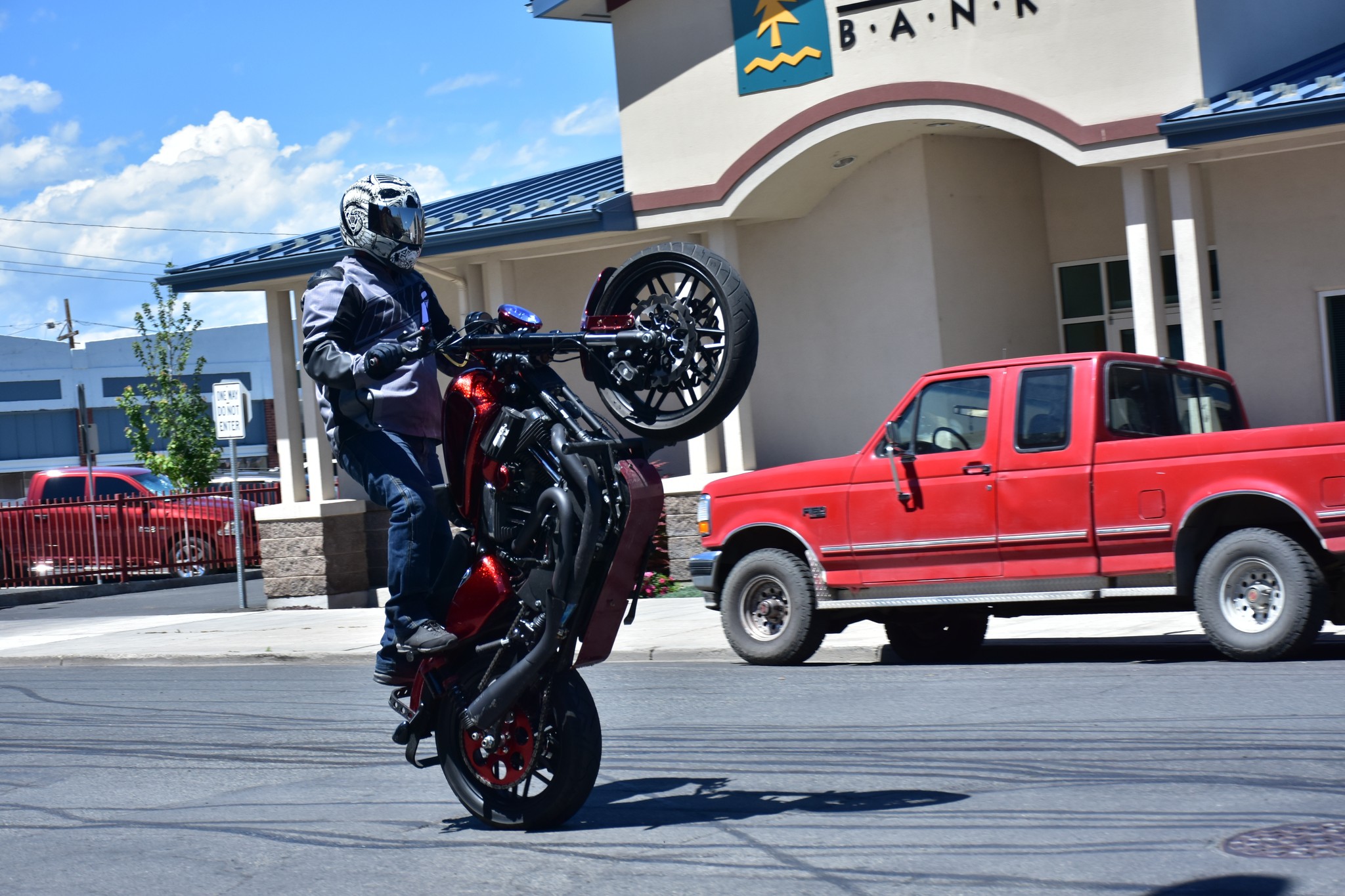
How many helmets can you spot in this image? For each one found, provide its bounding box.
[339,174,423,268]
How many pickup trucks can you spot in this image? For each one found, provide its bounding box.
[683,349,1345,664]
[0,466,273,582]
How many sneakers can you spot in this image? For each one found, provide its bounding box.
[373,668,415,686]
[396,621,458,653]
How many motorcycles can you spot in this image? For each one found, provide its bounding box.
[387,238,761,833]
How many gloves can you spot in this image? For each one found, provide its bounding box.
[365,341,407,381]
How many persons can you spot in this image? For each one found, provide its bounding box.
[299,173,493,690]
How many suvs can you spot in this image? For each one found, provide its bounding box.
[183,467,339,507]
